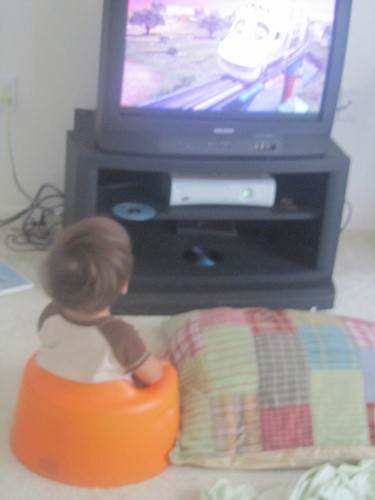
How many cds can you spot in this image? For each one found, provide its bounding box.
[184,245,224,267]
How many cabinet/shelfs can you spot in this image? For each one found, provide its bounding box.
[61,107,351,317]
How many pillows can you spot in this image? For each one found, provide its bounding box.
[165,307,374,467]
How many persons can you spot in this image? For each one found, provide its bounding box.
[33,217,172,390]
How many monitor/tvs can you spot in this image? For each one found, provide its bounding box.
[95,0,352,158]
